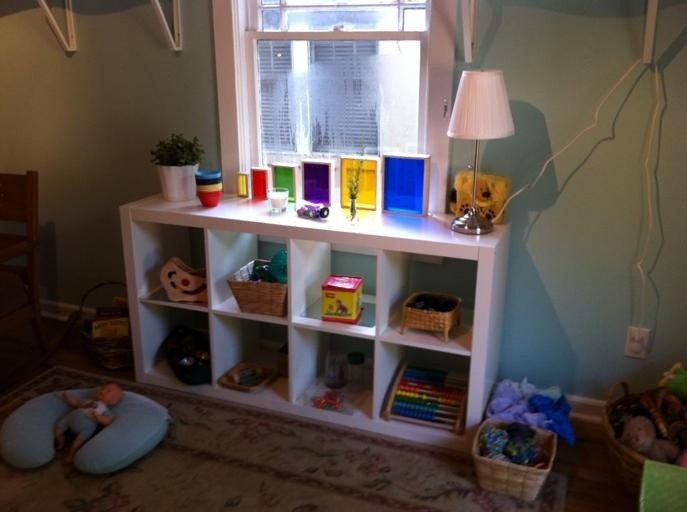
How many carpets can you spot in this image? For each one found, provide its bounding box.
[0,367,569,512]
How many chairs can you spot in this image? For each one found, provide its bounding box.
[1,170,55,393]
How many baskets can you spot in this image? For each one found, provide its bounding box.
[602,381,687,497]
[78,282,133,369]
[472,419,558,501]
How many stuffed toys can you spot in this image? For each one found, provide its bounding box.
[654,362,687,395]
[623,416,679,462]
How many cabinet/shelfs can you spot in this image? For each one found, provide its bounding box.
[120,190,512,455]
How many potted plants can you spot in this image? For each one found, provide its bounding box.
[149,134,206,201]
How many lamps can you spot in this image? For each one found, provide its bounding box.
[446,70,516,235]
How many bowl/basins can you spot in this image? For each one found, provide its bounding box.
[195,171,223,207]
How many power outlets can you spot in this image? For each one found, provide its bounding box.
[624,326,651,360]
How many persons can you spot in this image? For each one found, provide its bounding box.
[55,382,124,462]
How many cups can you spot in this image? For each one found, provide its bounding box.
[324,354,347,388]
[267,188,289,214]
